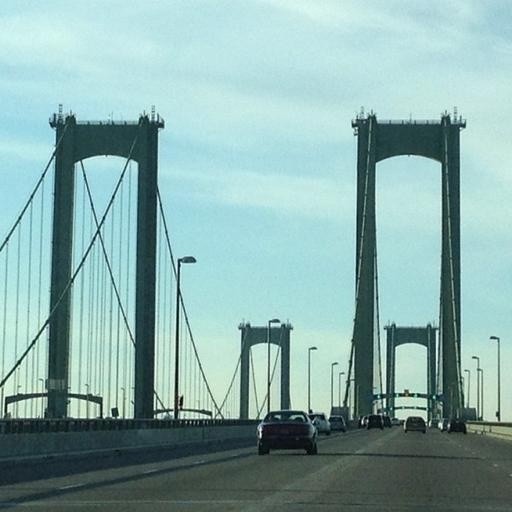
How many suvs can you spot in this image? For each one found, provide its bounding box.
[306,412,332,435]
[326,415,346,432]
[358,414,400,430]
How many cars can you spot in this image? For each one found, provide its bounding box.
[402,416,426,434]
[428,418,440,428]
[256,409,318,454]
[446,419,467,434]
[437,417,448,432]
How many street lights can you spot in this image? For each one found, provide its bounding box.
[307,347,317,412]
[463,369,470,409]
[330,362,339,409]
[173,256,197,421]
[471,355,479,421]
[489,335,500,422]
[14,379,158,422]
[347,378,356,415]
[266,317,280,414]
[476,369,483,421]
[338,371,345,407]
